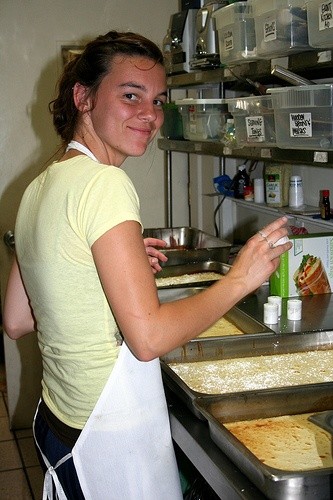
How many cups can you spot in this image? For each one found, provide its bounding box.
[253,179,264,203]
[264,304,278,324]
[287,300,301,321]
[268,296,282,316]
[288,175,304,208]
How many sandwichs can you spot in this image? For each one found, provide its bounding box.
[294,253,331,296]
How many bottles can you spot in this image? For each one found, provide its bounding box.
[233,164,250,199]
[162,28,172,74]
[320,191,331,221]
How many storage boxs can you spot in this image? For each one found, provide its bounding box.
[161,0,333,151]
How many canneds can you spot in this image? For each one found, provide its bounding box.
[264,161,289,207]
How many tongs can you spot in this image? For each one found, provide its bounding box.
[270,64,317,85]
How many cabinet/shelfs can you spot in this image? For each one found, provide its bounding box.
[158,48,333,167]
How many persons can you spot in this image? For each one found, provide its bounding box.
[0,30,295,499]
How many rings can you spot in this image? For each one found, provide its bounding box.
[264,237,273,247]
[257,230,266,238]
